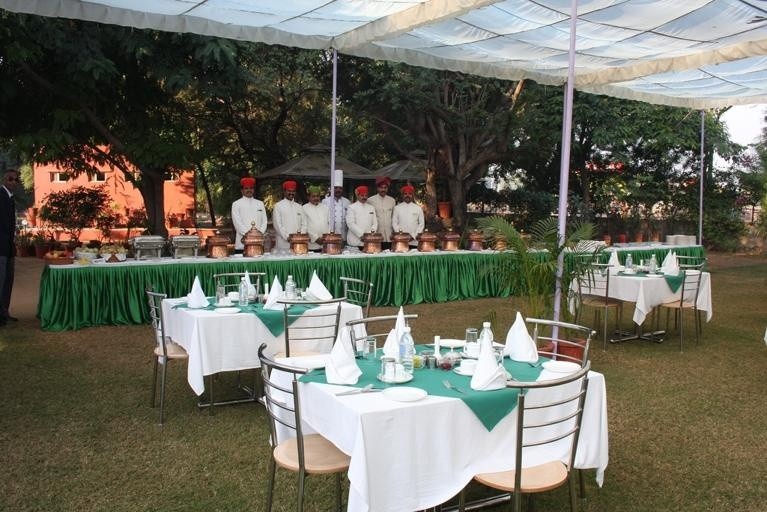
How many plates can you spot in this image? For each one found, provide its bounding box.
[379,386,427,402]
[378,373,414,384]
[437,338,465,349]
[272,353,332,371]
[539,360,582,374]
[188,294,243,314]
[454,366,473,376]
[623,271,638,275]
[646,274,664,278]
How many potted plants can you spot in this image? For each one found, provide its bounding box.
[480,211,597,359]
[538,241,610,362]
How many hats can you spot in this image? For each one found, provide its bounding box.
[240,177,256,186]
[334,169,344,188]
[306,186,320,194]
[401,185,416,193]
[283,181,297,190]
[355,186,368,195]
[376,176,391,187]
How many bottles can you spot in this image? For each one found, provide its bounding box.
[626,254,633,268]
[650,255,657,274]
[239,277,251,306]
[399,326,415,374]
[478,321,494,356]
[285,275,295,301]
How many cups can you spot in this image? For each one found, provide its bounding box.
[427,356,437,369]
[380,357,397,386]
[461,359,476,371]
[466,327,478,344]
[493,347,505,366]
[363,338,377,360]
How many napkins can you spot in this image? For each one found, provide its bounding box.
[500,311,538,363]
[325,328,363,384]
[383,306,417,358]
[471,329,508,391]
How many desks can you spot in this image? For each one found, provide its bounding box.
[32,240,707,333]
[258,338,611,511]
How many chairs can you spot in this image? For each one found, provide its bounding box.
[457,359,592,511]
[144,271,376,426]
[345,314,419,352]
[257,342,353,511]
[526,317,596,368]
[276,296,348,358]
[575,249,714,352]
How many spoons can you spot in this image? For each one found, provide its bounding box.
[335,383,374,396]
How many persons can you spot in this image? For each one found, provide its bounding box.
[230,175,269,257]
[301,184,332,253]
[366,175,396,251]
[390,184,426,250]
[1,169,19,327]
[321,186,351,249]
[271,179,309,250]
[345,185,378,252]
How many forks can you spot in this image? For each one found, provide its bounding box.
[439,379,467,395]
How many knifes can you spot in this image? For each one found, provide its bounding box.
[336,389,383,397]
